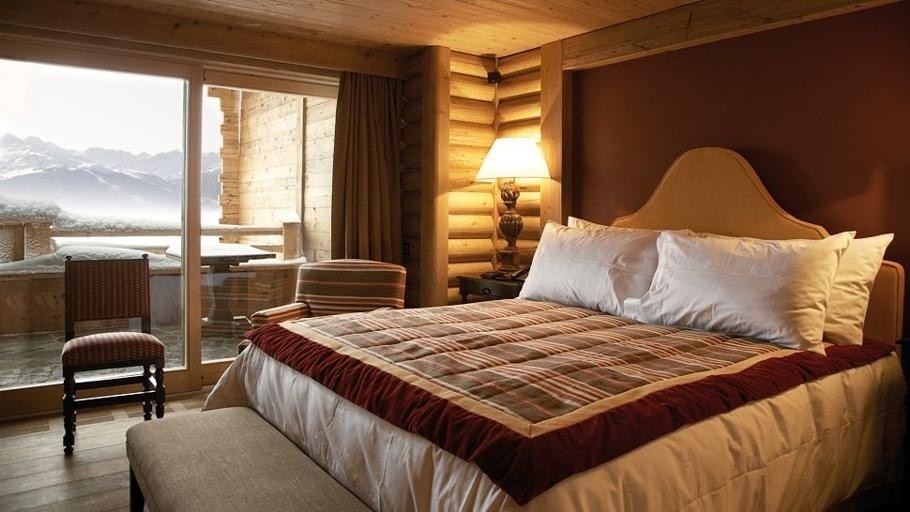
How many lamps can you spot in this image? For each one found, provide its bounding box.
[474,137,552,281]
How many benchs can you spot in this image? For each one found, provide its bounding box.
[123,407,376,511]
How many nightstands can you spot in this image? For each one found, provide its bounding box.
[456,274,527,302]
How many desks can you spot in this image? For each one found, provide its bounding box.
[166,242,276,337]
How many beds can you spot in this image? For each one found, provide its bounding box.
[243,146,904,512]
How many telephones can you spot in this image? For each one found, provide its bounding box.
[511,267,530,282]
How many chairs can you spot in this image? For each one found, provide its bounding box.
[62,254,166,459]
[236,258,407,356]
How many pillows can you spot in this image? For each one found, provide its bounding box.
[518,215,693,320]
[624,229,893,357]
